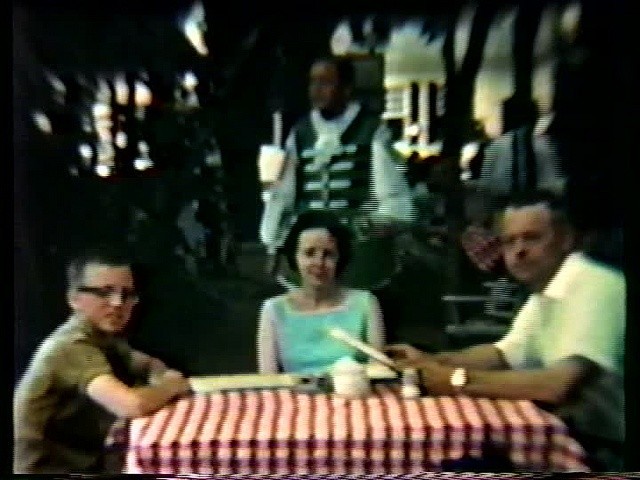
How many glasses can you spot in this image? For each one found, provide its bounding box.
[75,282,141,301]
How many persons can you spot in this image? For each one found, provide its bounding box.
[13,252,191,474]
[255,211,385,375]
[259,55,420,359]
[375,189,627,472]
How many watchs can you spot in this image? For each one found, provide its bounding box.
[449,367,469,397]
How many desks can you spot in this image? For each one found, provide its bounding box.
[123,382,591,480]
